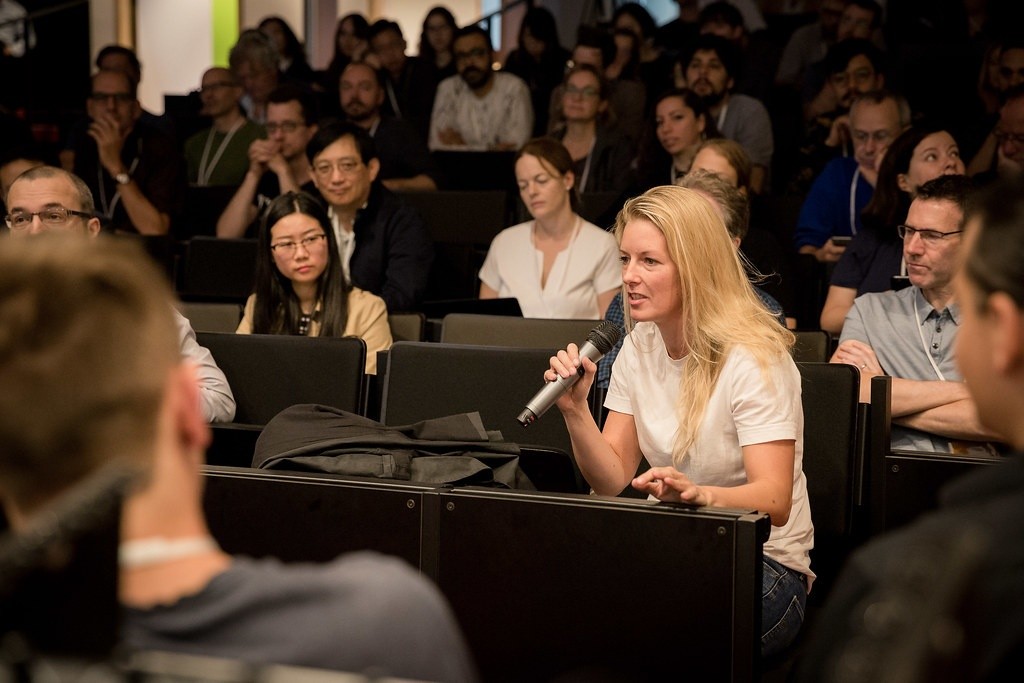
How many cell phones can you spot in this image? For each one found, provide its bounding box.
[832,236,852,246]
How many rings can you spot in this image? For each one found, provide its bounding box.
[860,364,866,370]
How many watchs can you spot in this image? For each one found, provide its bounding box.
[114,173,132,185]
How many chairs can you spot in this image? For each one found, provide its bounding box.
[130,64,1011,683]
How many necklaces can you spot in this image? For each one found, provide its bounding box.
[118,536,215,566]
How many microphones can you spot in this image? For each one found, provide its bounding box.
[516,321,623,427]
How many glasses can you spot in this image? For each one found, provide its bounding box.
[90,92,135,107]
[563,85,599,99]
[897,224,965,244]
[264,120,307,134]
[197,82,236,95]
[5,206,94,232]
[269,233,328,261]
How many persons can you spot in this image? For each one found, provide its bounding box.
[59,0,1024,389]
[795,174,1024,683]
[5,166,236,422]
[829,173,1005,458]
[0,226,471,683]
[544,184,815,650]
